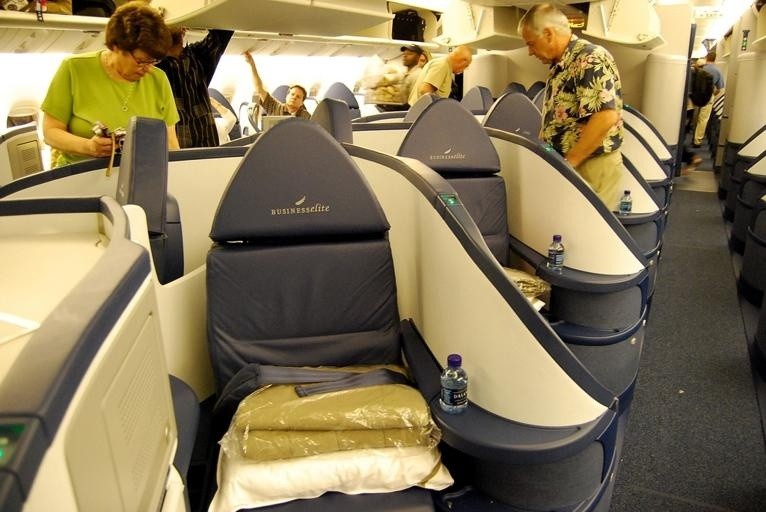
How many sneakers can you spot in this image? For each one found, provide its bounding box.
[692,142,701,149]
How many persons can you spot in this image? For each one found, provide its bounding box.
[153,25,220,149]
[681,52,725,171]
[517,2,624,213]
[207,87,241,141]
[40,0,181,169]
[240,49,312,122]
[375,45,472,112]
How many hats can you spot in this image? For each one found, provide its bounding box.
[400,43,424,56]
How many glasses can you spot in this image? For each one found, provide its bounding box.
[129,49,163,66]
[419,58,426,62]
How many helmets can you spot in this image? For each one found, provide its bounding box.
[695,57,706,67]
[422,47,433,62]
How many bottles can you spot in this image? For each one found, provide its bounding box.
[619,189,634,217]
[546,234,565,276]
[438,353,468,415]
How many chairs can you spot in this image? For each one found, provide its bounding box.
[104,83,675,512]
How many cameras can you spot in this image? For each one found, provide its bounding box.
[112,128,127,147]
[91,120,108,138]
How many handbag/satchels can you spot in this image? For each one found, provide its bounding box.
[391,7,427,42]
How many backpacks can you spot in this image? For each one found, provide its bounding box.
[689,68,715,109]
